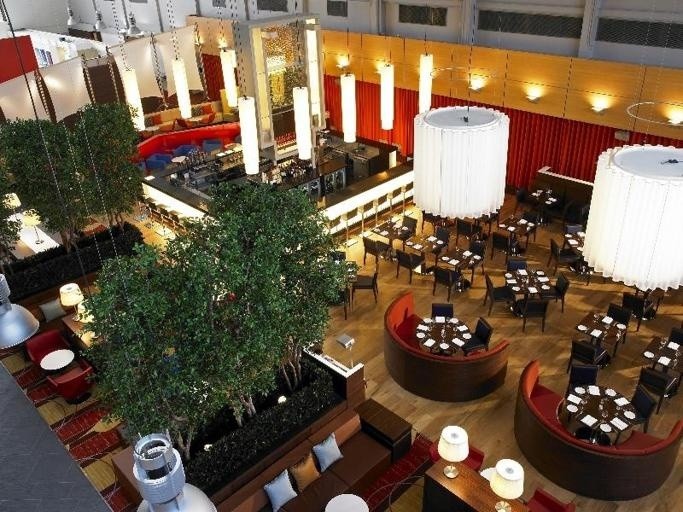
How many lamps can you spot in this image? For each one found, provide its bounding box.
[0,1,217,512]
[2,193,23,230]
[23,209,44,244]
[78,299,101,337]
[490,459,524,512]
[438,425,469,479]
[0,273,40,349]
[60,283,84,322]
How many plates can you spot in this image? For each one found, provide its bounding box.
[375,215,585,295]
[577,311,628,340]
[642,333,680,369]
[417,316,471,352]
[564,384,636,433]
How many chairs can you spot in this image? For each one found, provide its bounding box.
[526,488,576,512]
[463,446,485,472]
[47,359,96,405]
[25,328,70,376]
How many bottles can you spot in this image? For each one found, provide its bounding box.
[173,147,209,167]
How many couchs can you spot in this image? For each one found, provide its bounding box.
[514,360,683,501]
[217,398,412,512]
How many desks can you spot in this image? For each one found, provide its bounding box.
[40,349,75,375]
[325,494,369,512]
[425,458,531,512]
[61,313,109,352]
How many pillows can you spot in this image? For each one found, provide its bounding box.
[39,298,66,323]
[263,433,344,512]
